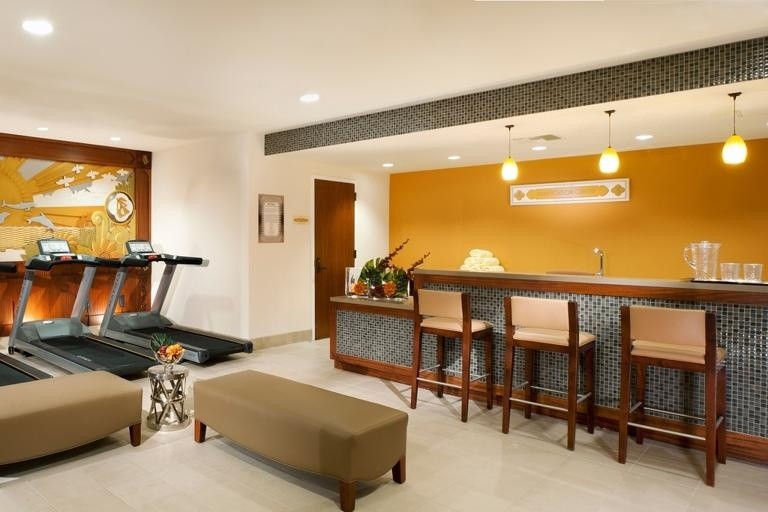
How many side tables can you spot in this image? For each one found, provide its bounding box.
[148,365,190,433]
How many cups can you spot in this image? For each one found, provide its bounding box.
[744,264,762,283]
[720,263,738,282]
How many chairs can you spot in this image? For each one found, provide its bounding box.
[501,296,596,450]
[411,288,494,422]
[618,305,726,488]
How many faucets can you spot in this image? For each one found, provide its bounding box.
[595,249,604,275]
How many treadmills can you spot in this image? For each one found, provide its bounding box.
[7,238,162,375]
[97,240,253,365]
[0,250,54,387]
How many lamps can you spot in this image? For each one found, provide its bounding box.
[722,93,748,166]
[501,125,519,181]
[599,110,620,174]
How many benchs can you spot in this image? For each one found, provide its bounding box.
[0,371,143,466]
[193,369,408,512]
